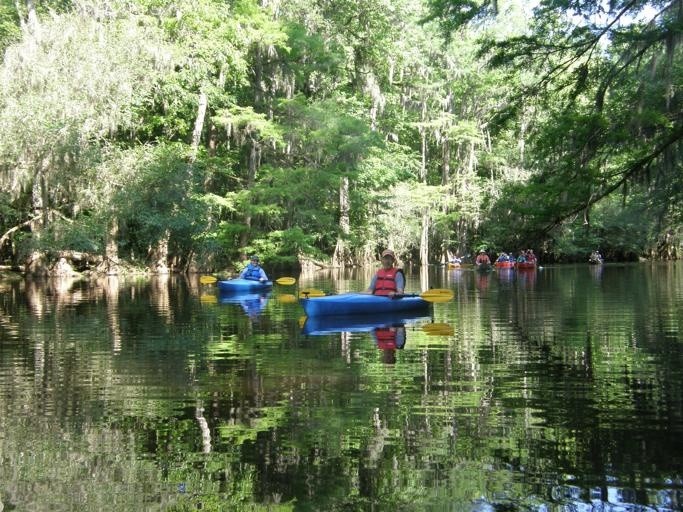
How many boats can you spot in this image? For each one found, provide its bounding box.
[218,278,272,292]
[473,258,538,272]
[447,262,460,268]
[216,293,274,314]
[297,293,429,317]
[589,257,601,264]
[298,314,430,336]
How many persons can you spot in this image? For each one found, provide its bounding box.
[589,250,603,265]
[448,256,462,263]
[475,249,537,264]
[240,256,269,283]
[366,249,405,299]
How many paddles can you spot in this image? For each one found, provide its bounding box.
[200,276,296,286]
[299,288,454,303]
[404,323,457,337]
[202,294,297,303]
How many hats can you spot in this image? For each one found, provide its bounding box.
[252,256,259,260]
[382,249,394,258]
[479,249,485,253]
